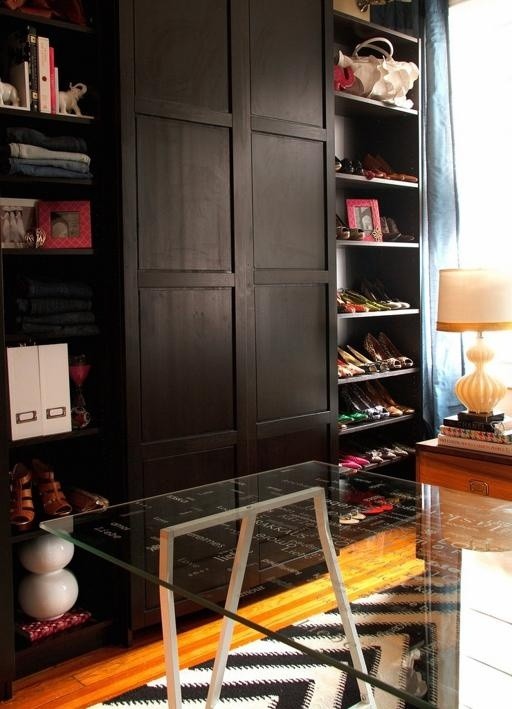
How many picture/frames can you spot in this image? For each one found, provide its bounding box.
[346,199,382,242]
[37,201,93,249]
[0,198,40,249]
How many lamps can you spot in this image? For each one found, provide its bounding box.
[437,269,512,423]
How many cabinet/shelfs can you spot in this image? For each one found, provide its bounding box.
[332,8,426,548]
[0,8,132,702]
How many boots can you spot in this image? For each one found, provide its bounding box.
[380,216,415,242]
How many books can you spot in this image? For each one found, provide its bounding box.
[0,24,61,115]
[443,411,512,433]
[437,434,512,457]
[438,424,512,445]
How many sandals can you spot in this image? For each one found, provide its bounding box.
[334,332,414,378]
[9,459,73,526]
[336,215,364,240]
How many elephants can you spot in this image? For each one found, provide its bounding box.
[58,81,88,117]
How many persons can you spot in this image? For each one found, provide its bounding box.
[1,210,11,243]
[9,211,20,243]
[15,210,27,242]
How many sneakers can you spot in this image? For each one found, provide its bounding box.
[335,154,418,182]
[340,442,416,469]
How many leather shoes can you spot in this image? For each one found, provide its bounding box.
[336,277,411,313]
[338,380,416,425]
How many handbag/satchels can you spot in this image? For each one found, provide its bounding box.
[334,37,419,109]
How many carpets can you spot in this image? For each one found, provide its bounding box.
[88,574,458,709]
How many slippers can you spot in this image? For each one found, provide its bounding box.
[339,493,399,525]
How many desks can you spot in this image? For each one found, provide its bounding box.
[39,460,512,709]
[414,437,512,577]
